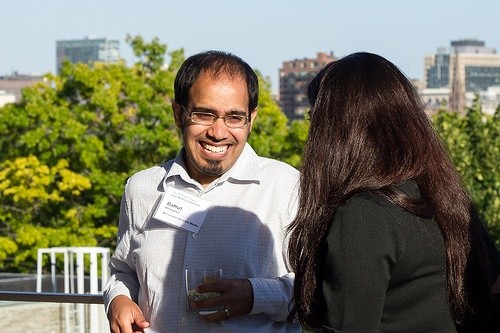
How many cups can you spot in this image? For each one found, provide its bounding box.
[185,270,223,311]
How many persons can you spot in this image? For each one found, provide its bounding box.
[284,52,500,333]
[103,50,303,333]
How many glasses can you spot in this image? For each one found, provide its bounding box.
[178,98,252,129]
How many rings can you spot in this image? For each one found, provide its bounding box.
[223,308,230,317]
